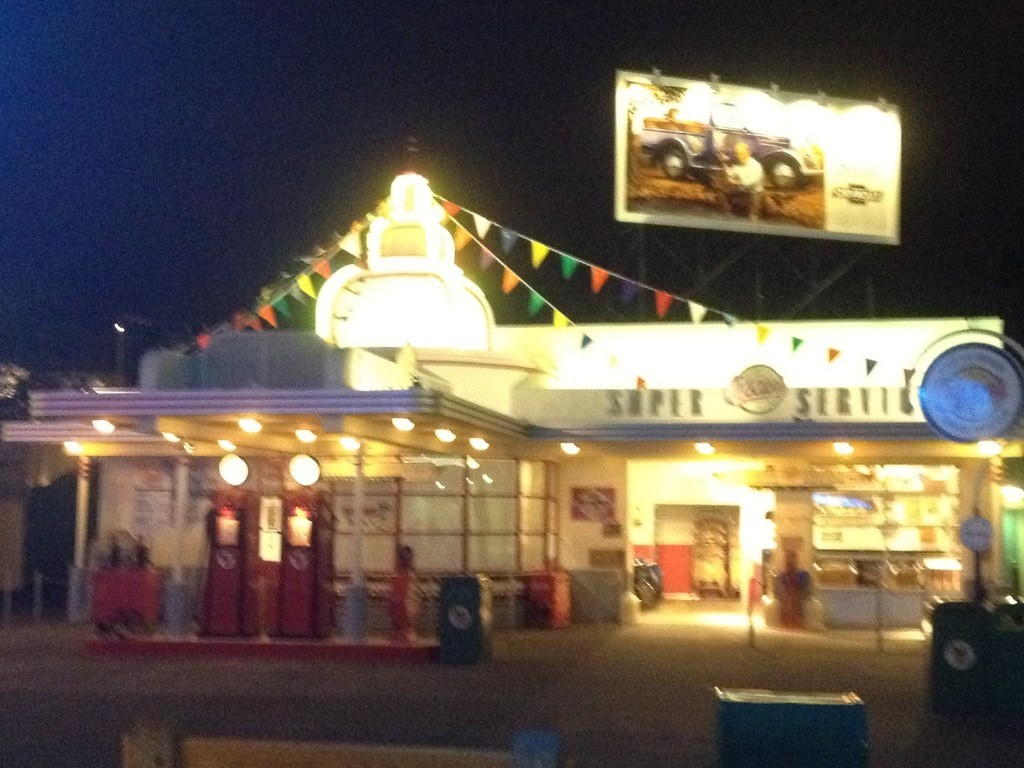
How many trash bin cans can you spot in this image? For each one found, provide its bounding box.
[927,602,993,714]
[438,574,492,667]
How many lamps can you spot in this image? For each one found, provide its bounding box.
[692,441,716,456]
[831,441,856,457]
[63,416,489,486]
[559,440,581,456]
